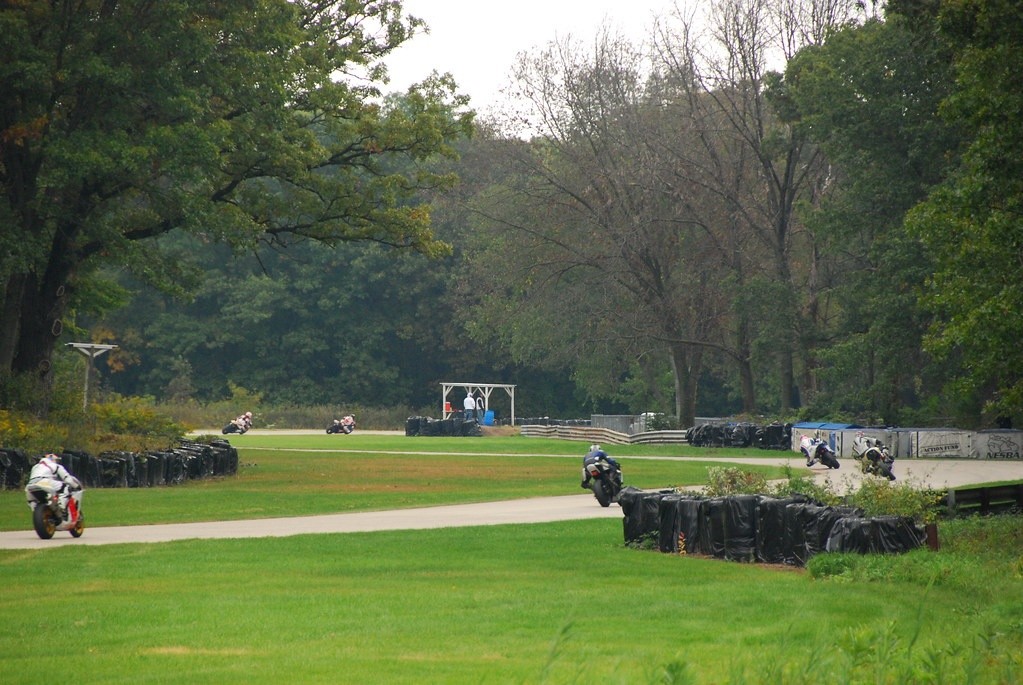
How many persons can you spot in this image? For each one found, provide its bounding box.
[800,435,836,457]
[25,454,81,512]
[476,393,484,425]
[852,430,889,463]
[463,393,475,420]
[239,412,252,429]
[581,444,619,487]
[342,414,355,433]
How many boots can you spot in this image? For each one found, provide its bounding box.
[28,500,37,511]
[57,497,69,516]
[813,458,819,463]
[614,469,622,486]
[825,445,835,454]
[583,483,593,489]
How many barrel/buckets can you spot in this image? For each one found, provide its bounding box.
[485,410,495,426]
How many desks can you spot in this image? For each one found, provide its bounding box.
[442,411,465,419]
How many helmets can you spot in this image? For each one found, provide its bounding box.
[589,445,600,450]
[855,430,864,437]
[45,454,61,464]
[246,412,252,419]
[351,414,355,418]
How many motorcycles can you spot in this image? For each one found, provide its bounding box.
[222,418,252,434]
[326,420,356,434]
[26,475,84,539]
[586,461,624,507]
[862,444,896,481]
[815,443,841,469]
